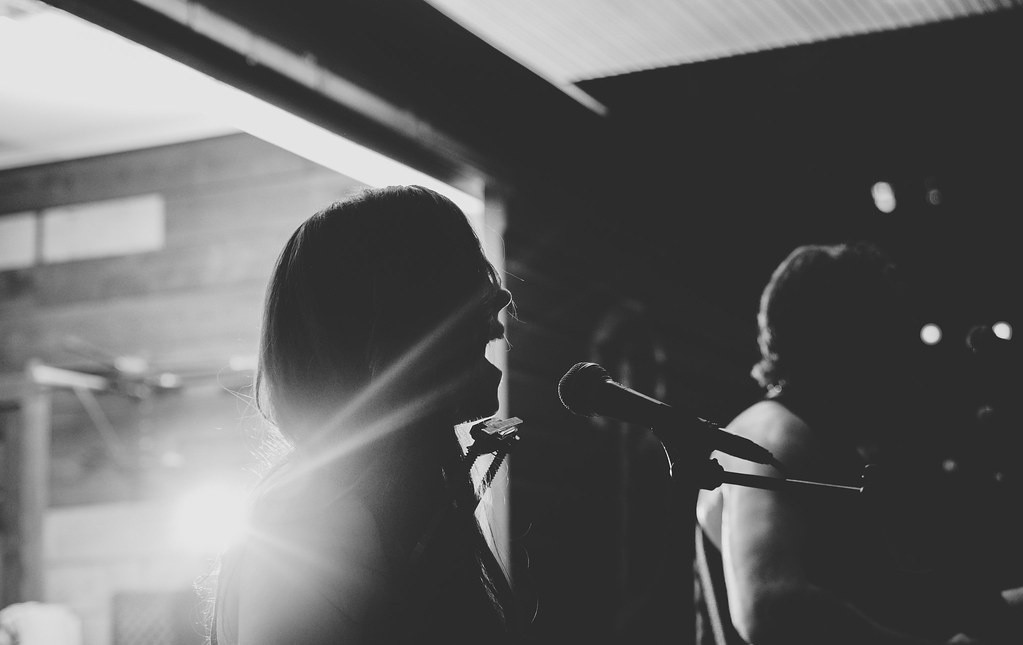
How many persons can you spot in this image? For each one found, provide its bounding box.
[210,186,519,644]
[694,241,1023,645]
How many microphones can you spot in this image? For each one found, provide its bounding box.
[557,362,776,465]
[967,325,999,357]
